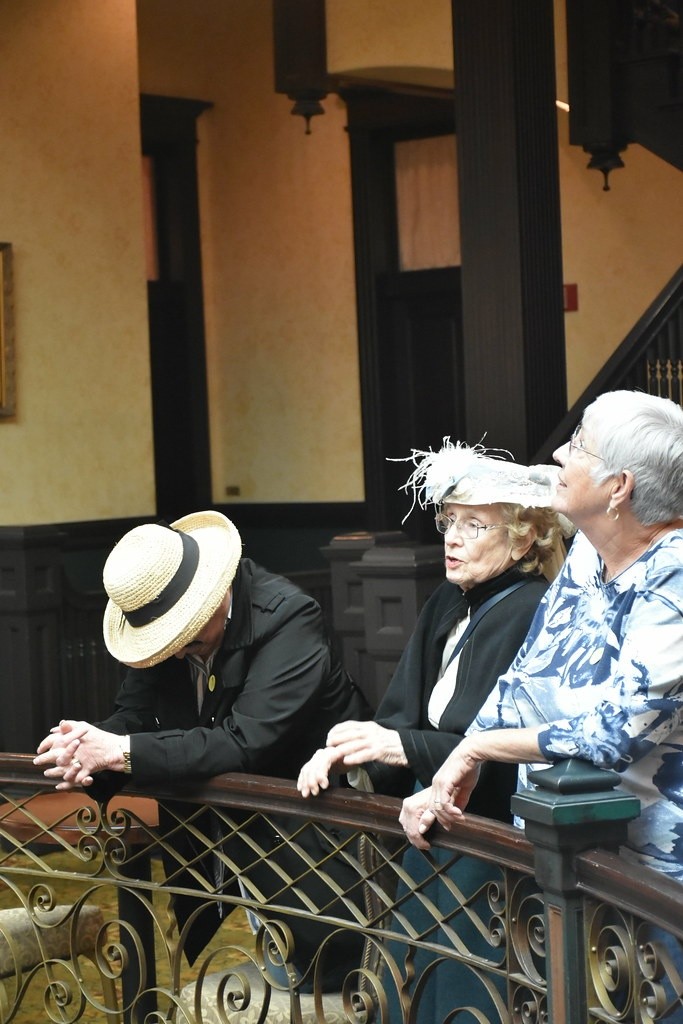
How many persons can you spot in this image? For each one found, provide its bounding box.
[399,390,683,1024]
[297,442,576,1024]
[33,511,375,991]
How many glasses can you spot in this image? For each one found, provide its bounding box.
[185,640,203,648]
[569,425,633,500]
[434,513,521,539]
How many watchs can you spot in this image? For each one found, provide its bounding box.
[122,735,132,773]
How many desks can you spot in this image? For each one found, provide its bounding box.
[0,792,160,1024]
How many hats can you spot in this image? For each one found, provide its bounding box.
[102,509,243,669]
[386,434,577,540]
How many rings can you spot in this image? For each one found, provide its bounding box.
[73,760,80,764]
[434,800,439,803]
